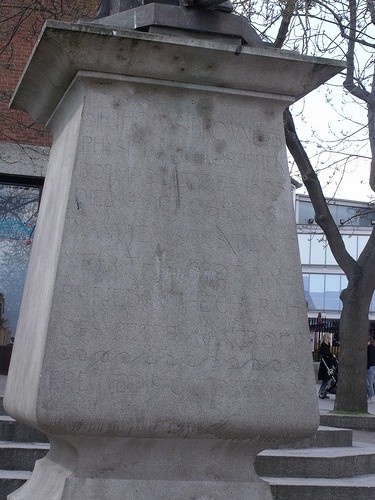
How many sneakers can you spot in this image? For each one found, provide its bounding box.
[367,395,373,403]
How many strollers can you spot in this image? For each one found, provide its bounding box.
[320,354,339,399]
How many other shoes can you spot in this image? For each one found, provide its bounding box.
[319,394,330,399]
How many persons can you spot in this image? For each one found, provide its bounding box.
[365,335,375,406]
[317,333,338,401]
